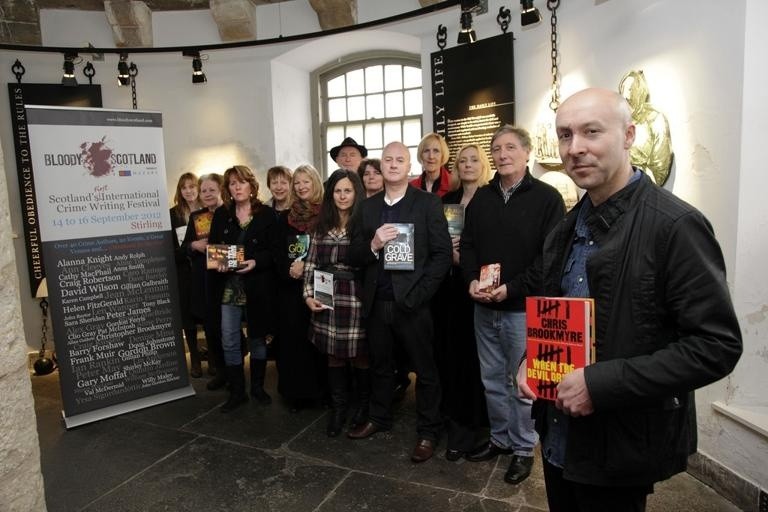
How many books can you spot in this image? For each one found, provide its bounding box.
[443,203,465,239]
[524,296,591,402]
[477,262,501,293]
[538,296,596,366]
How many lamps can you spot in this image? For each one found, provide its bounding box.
[54,43,211,92]
[449,1,544,45]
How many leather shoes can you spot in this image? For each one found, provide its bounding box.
[190,366,202,377]
[348,420,385,439]
[465,441,513,462]
[504,456,534,484]
[411,438,434,461]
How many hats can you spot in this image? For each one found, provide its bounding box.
[330,137,368,161]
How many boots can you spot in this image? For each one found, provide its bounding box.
[250,358,271,403]
[207,359,248,412]
[352,374,372,426]
[325,366,345,437]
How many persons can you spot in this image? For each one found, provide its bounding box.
[348,142,453,465]
[515,86,743,512]
[205,168,273,414]
[460,125,568,486]
[440,143,490,461]
[409,132,454,197]
[329,137,368,174]
[170,160,384,375]
[304,170,367,438]
